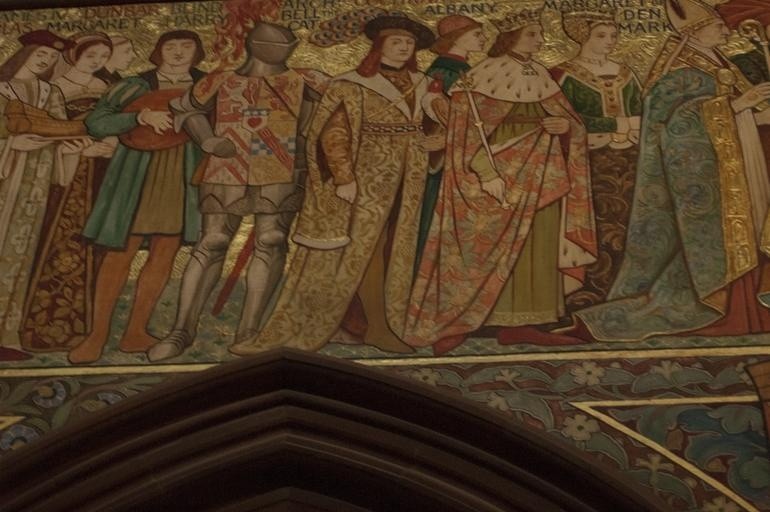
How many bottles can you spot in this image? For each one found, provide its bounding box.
[118,88,191,152]
[664,1,722,38]
[17,30,114,66]
[363,9,616,51]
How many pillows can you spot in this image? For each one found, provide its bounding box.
[244,22,300,65]
